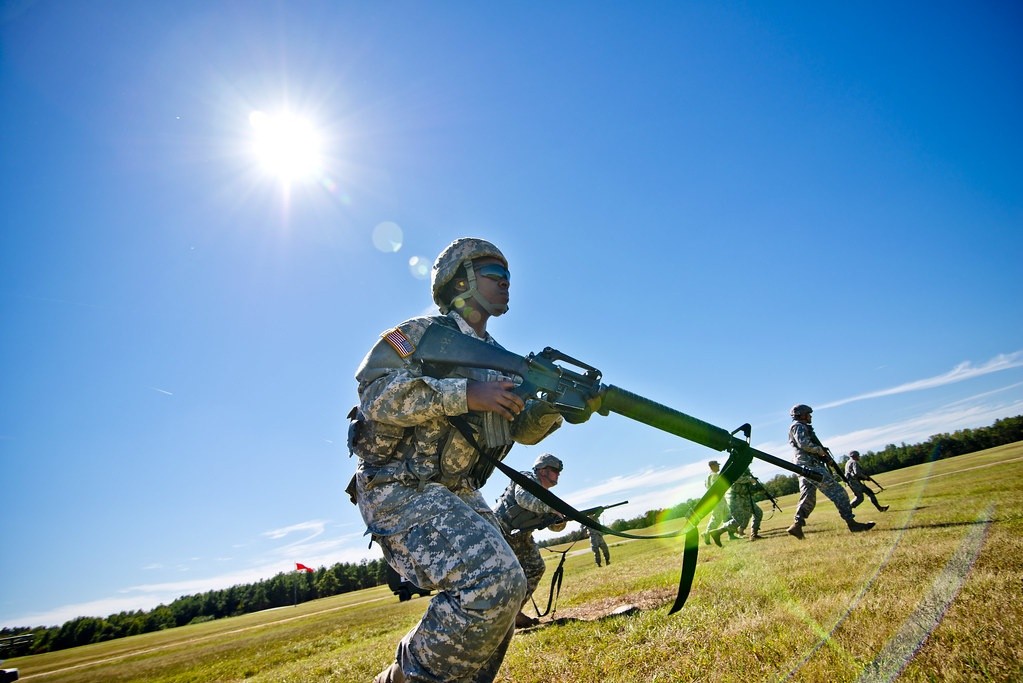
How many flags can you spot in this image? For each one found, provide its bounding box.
[296,563,313,572]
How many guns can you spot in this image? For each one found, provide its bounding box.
[808,430,850,487]
[863,474,885,490]
[748,475,784,514]
[509,501,629,535]
[412,323,826,485]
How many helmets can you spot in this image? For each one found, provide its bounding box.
[431,238,508,306]
[850,451,859,457]
[531,454,562,470]
[790,404,813,417]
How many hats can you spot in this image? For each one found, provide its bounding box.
[709,461,720,466]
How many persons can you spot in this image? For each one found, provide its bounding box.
[348,238,563,683]
[492,454,568,629]
[845,451,889,513]
[786,405,877,540]
[701,458,763,548]
[586,509,610,569]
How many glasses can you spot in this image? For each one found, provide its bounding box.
[472,264,510,282]
[542,468,560,474]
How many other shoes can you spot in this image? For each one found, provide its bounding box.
[374,660,407,683]
[878,505,889,512]
[846,519,876,532]
[701,530,712,546]
[730,532,743,540]
[786,522,805,540]
[597,563,601,567]
[739,527,746,536]
[606,560,610,565]
[750,528,761,541]
[515,611,539,629]
[709,525,727,547]
